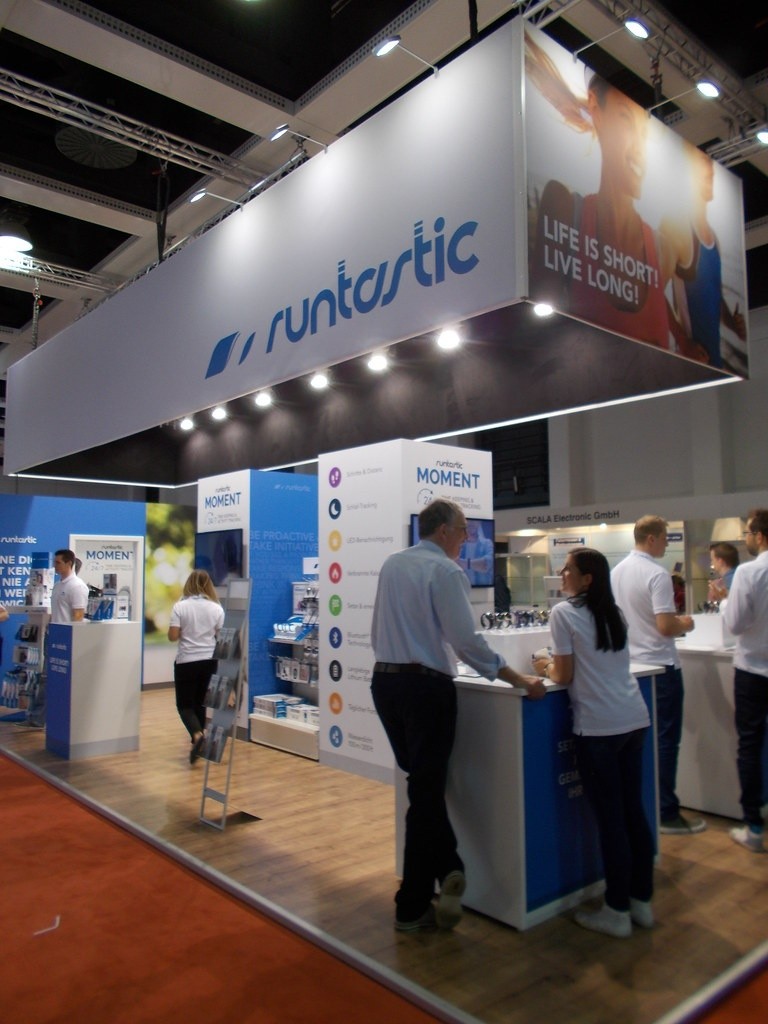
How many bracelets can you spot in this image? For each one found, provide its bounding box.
[544,662,551,677]
[467,558,470,569]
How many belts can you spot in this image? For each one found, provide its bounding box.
[373,662,452,680]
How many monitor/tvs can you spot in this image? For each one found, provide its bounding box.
[410,513,495,587]
[195,529,243,585]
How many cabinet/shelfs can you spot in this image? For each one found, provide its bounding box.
[1,606,49,728]
[249,579,320,761]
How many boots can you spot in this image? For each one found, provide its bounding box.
[632,899,654,927]
[575,902,632,938]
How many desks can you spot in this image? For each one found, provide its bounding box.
[395,613,743,932]
[45,620,142,760]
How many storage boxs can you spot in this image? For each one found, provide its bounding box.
[253,694,320,726]
[25,551,55,605]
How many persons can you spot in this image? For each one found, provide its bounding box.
[534,65,669,349]
[611,514,705,834]
[710,542,739,590]
[455,519,493,585]
[661,140,747,371]
[709,509,768,852]
[371,499,546,930]
[51,549,89,622]
[671,575,685,612]
[168,568,225,764]
[532,548,655,936]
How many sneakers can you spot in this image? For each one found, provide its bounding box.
[660,816,706,834]
[730,825,764,851]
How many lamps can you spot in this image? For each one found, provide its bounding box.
[0,221,33,251]
[573,18,650,63]
[709,130,768,156]
[648,80,721,120]
[188,188,243,206]
[269,124,328,148]
[372,35,438,74]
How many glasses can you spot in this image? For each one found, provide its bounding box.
[449,522,470,535]
[741,530,760,539]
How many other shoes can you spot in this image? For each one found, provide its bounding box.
[395,902,436,930]
[435,871,467,930]
[189,732,205,763]
